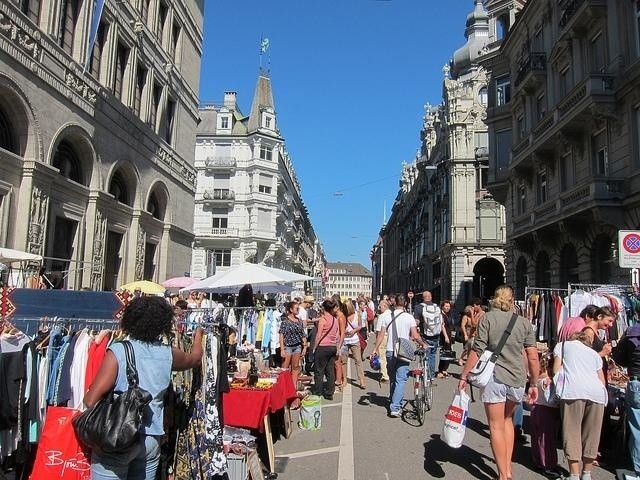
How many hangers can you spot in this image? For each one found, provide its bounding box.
[2,306,272,338]
[514,282,632,306]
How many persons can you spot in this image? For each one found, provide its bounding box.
[175,300,186,313]
[553,331,608,479]
[529,350,562,473]
[372,296,427,417]
[457,288,540,480]
[559,305,640,478]
[83,295,204,480]
[277,292,457,389]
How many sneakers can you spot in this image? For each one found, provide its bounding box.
[391,412,402,418]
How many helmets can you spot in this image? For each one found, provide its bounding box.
[370,355,381,370]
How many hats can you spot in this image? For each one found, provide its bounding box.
[304,296,315,302]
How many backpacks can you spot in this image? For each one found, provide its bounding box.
[420,303,442,336]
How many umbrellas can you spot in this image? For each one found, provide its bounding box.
[179,258,316,301]
[120,279,167,295]
[161,275,199,291]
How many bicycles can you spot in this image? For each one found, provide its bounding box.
[407,345,439,425]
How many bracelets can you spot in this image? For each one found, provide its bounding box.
[460,378,467,382]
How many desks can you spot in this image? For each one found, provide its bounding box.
[608,379,628,465]
[223,371,299,473]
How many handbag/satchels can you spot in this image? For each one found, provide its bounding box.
[466,349,499,388]
[357,332,367,350]
[553,366,564,405]
[29,406,92,480]
[439,349,456,361]
[393,338,415,363]
[306,347,314,363]
[299,394,325,431]
[72,387,152,457]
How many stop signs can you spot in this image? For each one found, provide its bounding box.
[408,292,413,298]
